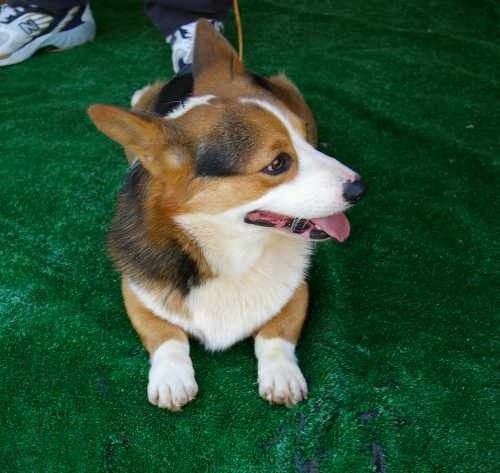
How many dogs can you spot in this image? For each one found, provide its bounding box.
[88,16,367,413]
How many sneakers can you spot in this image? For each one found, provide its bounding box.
[166,18,223,72]
[0,0,97,67]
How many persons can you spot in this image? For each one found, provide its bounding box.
[0,0,231,77]
